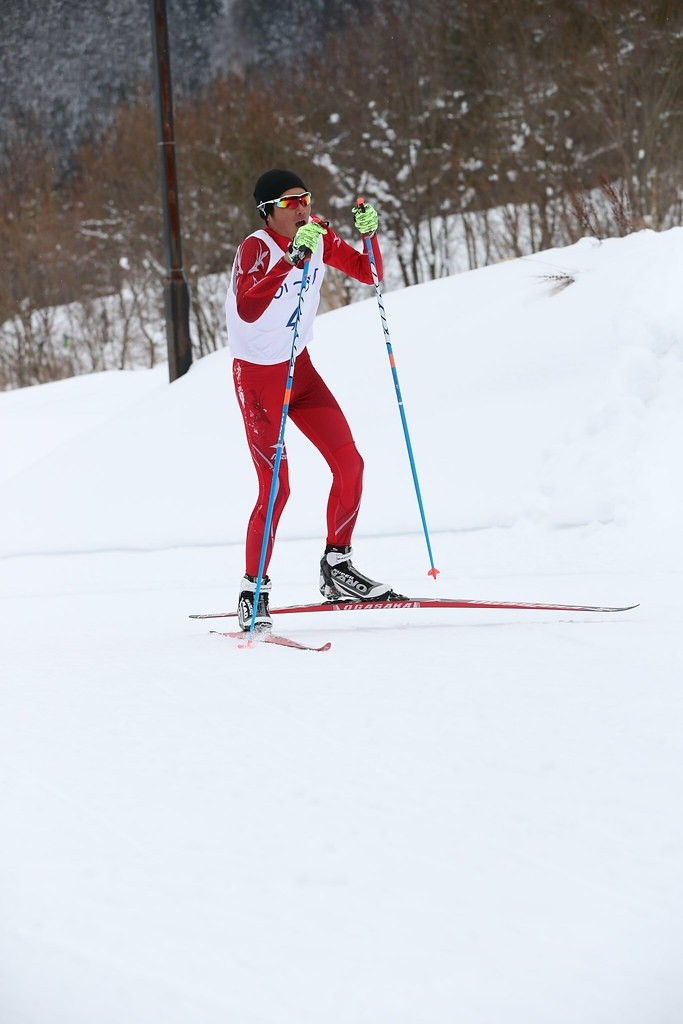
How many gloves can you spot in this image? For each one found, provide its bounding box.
[287,223,327,265]
[351,204,378,234]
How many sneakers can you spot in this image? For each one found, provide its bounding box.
[237,573,272,633]
[318,545,394,601]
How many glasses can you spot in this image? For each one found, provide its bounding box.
[256,192,311,210]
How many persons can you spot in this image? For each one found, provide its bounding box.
[226,169,408,631]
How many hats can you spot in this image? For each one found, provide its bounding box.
[254,169,308,218]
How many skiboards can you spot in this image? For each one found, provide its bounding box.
[186,596,641,652]
